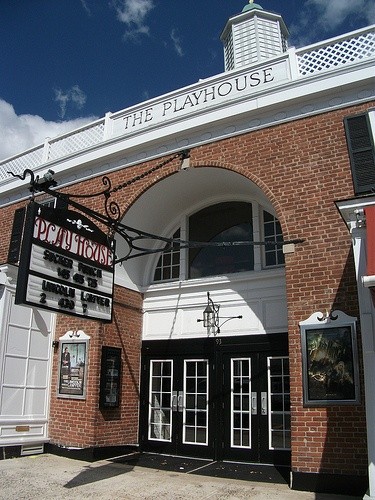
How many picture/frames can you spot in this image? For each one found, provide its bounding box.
[56,330,91,400]
[298,309,361,408]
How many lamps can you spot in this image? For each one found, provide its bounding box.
[197,292,243,334]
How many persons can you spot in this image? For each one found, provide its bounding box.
[61,347,71,385]
